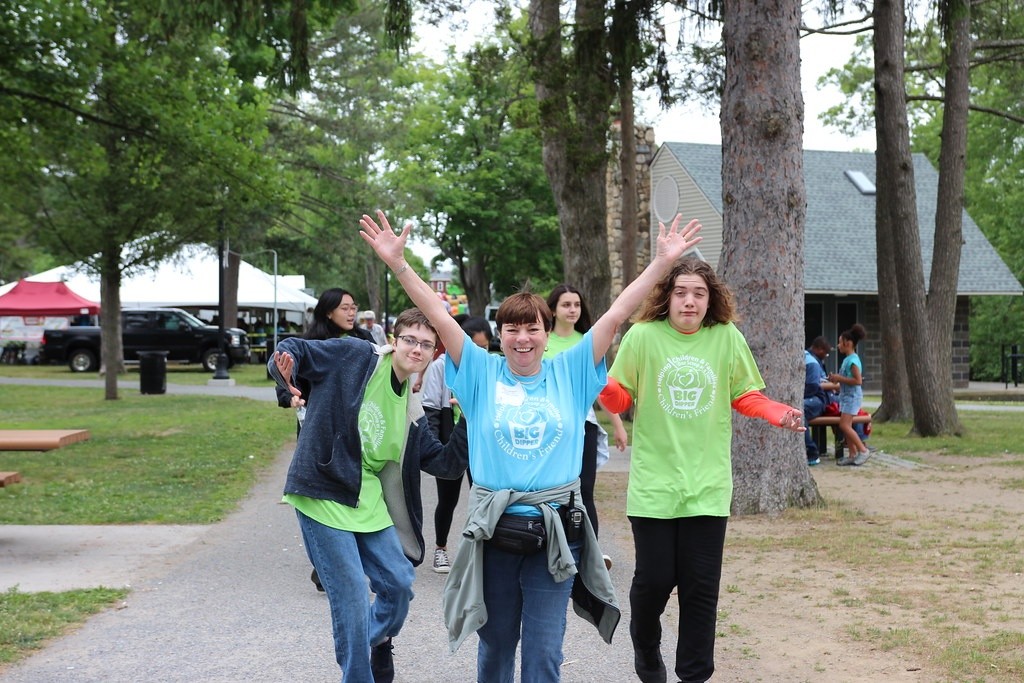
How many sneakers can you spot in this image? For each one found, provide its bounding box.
[370,635,395,683]
[433,549,451,572]
[630,621,667,683]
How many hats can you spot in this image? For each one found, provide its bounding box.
[363,310,376,320]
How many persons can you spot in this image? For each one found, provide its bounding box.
[359,310,388,347]
[421,316,493,572]
[808,335,878,452]
[437,292,451,313]
[542,284,609,537]
[268,308,469,683]
[601,256,807,683]
[255,317,296,344]
[296,287,377,595]
[828,323,871,466]
[358,210,704,683]
[804,349,828,466]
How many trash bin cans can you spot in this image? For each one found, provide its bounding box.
[136,350,170,396]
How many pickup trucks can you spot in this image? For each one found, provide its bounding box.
[40,308,251,375]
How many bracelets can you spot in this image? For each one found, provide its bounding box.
[394,263,409,275]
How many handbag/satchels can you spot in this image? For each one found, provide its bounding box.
[490,513,548,552]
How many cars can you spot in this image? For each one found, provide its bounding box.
[483,302,512,348]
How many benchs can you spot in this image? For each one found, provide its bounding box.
[807,415,872,461]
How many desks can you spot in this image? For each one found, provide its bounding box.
[0,428,90,454]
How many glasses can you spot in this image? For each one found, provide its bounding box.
[337,304,357,314]
[394,334,437,357]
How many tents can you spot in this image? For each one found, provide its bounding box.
[0,229,319,336]
[0,276,101,327]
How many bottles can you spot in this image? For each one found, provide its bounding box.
[295,405,306,428]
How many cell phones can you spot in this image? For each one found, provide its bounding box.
[562,491,585,542]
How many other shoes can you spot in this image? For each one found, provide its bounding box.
[854,447,872,465]
[807,457,821,466]
[837,457,855,465]
[602,555,611,570]
